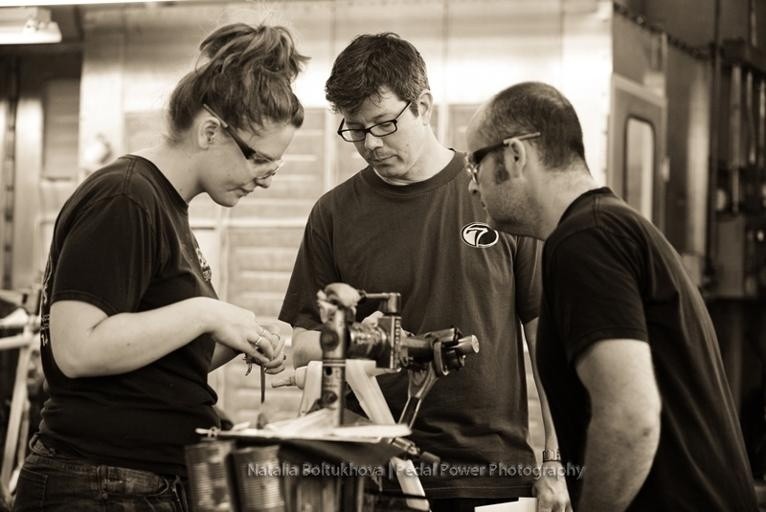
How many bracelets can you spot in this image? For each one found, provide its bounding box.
[542,449,562,462]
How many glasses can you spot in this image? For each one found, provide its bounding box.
[337,101,412,142]
[203,103,284,180]
[464,131,541,185]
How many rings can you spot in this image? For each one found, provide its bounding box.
[254,337,263,346]
[261,328,267,337]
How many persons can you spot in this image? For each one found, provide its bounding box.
[462,80,760,512]
[279,31,575,511]
[11,22,311,512]
